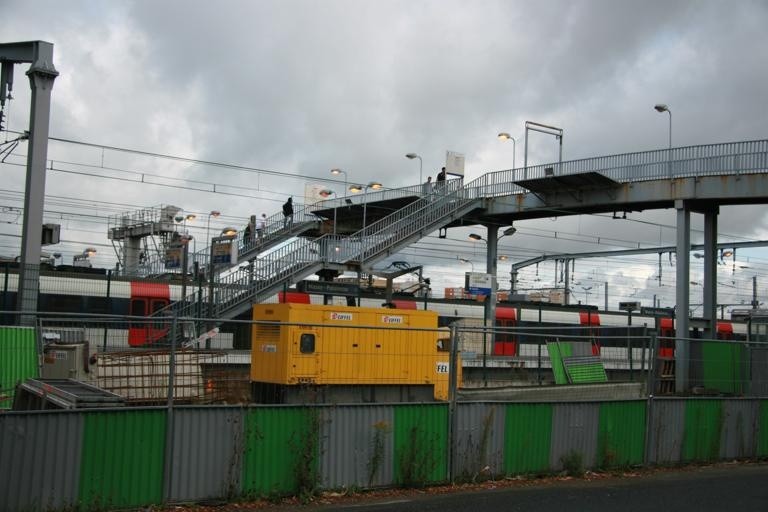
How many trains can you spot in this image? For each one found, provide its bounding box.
[1,274,767,371]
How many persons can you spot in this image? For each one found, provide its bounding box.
[423,176,436,201]
[243,222,253,246]
[435,167,447,197]
[282,196,294,230]
[257,213,266,242]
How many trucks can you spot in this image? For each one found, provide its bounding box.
[250,303,462,403]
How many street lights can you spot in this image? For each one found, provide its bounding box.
[320,189,336,259]
[83,248,97,268]
[53,253,62,265]
[206,210,220,315]
[582,287,591,304]
[695,251,731,258]
[406,151,423,195]
[184,214,197,282]
[498,131,515,193]
[218,228,236,319]
[330,167,347,197]
[655,103,672,177]
[348,181,382,277]
